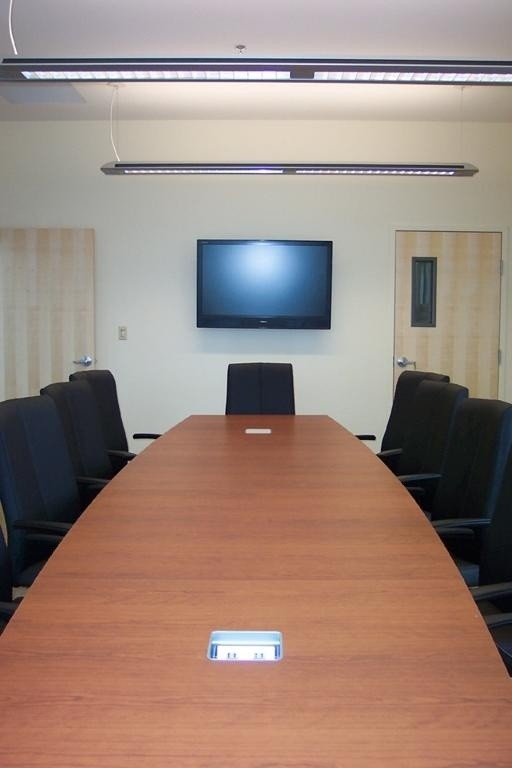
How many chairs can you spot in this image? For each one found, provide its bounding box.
[373,370,512,682]
[225,362,295,416]
[1,371,163,767]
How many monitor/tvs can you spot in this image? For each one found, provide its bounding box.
[196,240,332,329]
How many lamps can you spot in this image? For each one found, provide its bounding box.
[0,1,512,85]
[101,85,480,177]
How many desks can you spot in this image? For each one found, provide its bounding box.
[1,414,511,765]
